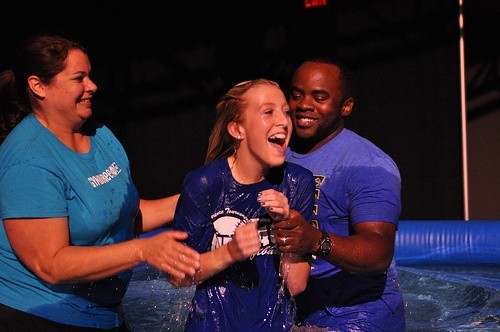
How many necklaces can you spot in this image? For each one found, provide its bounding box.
[231,149,264,184]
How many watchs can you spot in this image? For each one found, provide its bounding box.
[317,230,333,260]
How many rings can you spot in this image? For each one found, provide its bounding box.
[283,237,286,244]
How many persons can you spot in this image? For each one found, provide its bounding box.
[239,46,410,332]
[0,32,203,331]
[168,76,316,332]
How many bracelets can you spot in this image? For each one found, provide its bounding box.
[228,241,237,264]
